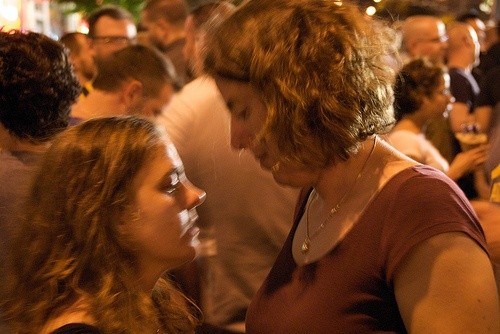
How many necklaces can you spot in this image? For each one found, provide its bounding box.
[302,134,377,253]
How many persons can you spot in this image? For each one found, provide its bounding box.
[157,74,302,333]
[0,25,83,334]
[386,56,491,183]
[398,6,500,200]
[61,0,237,127]
[0,115,207,334]
[190,1,500,334]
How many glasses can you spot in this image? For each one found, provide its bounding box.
[422,37,449,43]
[94,37,131,44]
